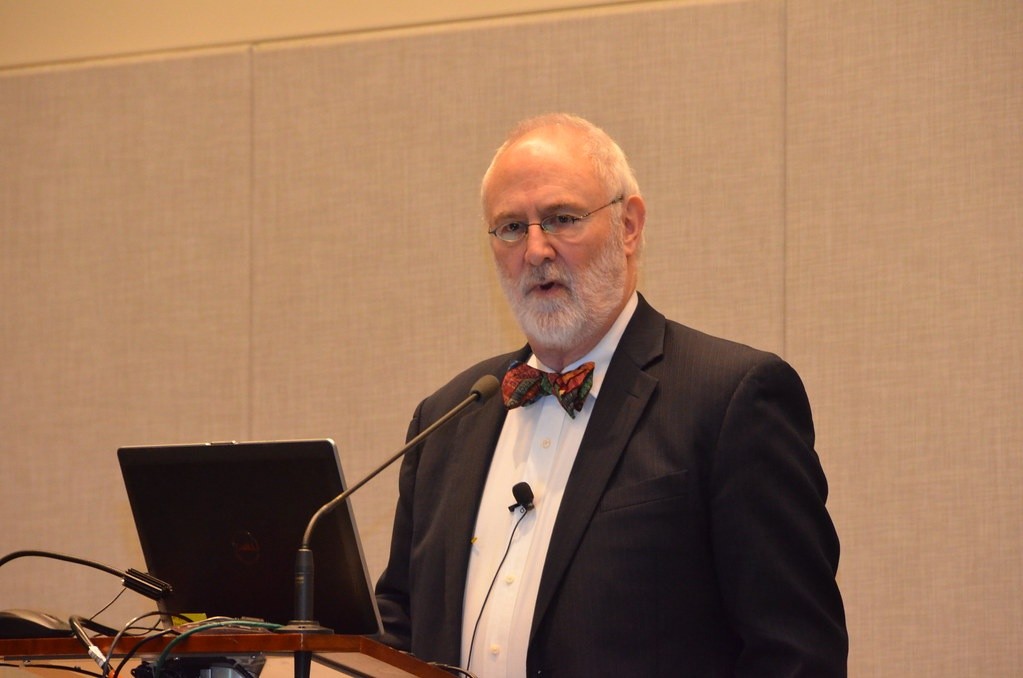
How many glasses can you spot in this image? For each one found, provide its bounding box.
[487,196,623,244]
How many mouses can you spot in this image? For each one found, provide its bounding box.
[0,608,73,639]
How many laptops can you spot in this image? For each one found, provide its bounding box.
[117,437,444,669]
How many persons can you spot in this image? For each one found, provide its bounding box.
[369,113,850,678]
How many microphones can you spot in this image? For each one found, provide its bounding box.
[512,483,535,511]
[0,550,174,603]
[276,375,501,634]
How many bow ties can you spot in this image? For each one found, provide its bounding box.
[502,360,595,418]
